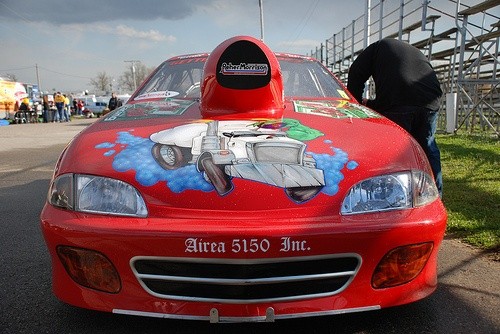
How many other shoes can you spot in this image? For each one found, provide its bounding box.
[52,119,71,123]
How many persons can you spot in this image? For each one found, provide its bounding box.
[347,38,444,202]
[109,92,118,111]
[9,90,83,125]
[117,98,123,108]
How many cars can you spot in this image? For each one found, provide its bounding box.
[81,101,109,115]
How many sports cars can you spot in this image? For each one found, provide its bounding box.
[39,36,449,323]
